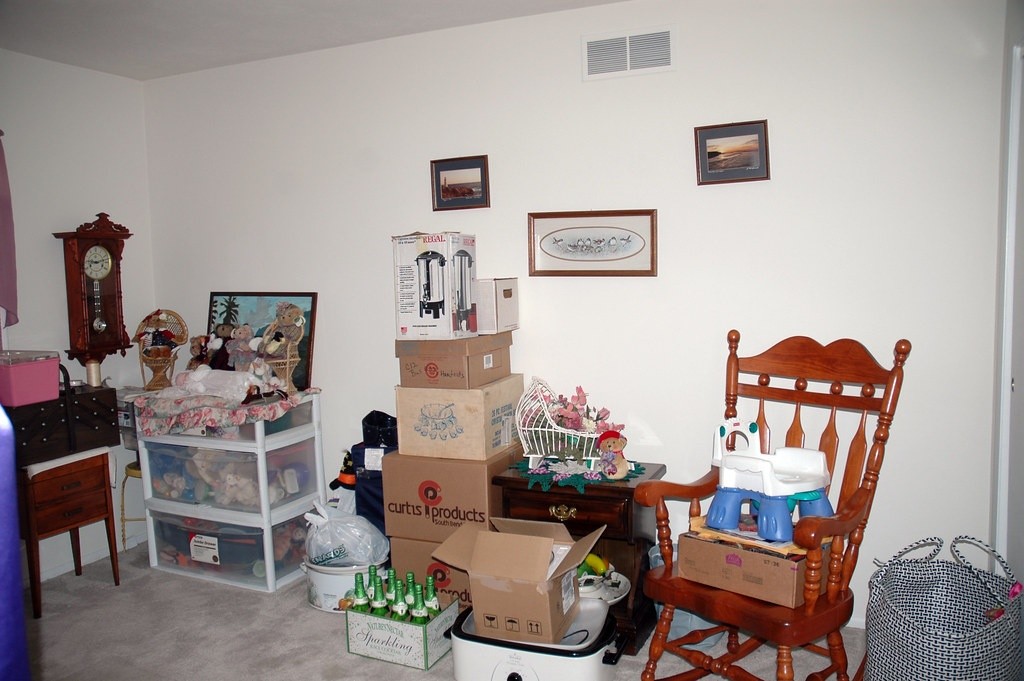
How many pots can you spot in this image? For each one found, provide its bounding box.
[299,557,389,614]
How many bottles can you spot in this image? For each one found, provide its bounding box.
[424,576,440,612]
[410,584,430,624]
[404,572,416,610]
[386,568,395,605]
[352,573,371,613]
[364,565,377,607]
[390,579,410,621]
[370,576,390,616]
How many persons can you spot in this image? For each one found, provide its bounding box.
[175,364,258,396]
[275,521,307,543]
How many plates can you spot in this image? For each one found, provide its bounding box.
[601,571,631,606]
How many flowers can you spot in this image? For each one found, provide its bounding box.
[518,384,625,467]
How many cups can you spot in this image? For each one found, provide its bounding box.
[86,360,101,387]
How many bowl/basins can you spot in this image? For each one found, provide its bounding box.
[578,575,606,598]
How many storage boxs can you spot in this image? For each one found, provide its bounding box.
[478,277,520,335]
[391,230,479,340]
[394,331,513,389]
[394,372,526,461]
[381,443,524,542]
[677,531,832,609]
[392,537,473,612]
[0,349,61,408]
[9,384,121,466]
[431,517,607,643]
[335,584,461,671]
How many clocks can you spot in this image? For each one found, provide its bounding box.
[51,212,134,366]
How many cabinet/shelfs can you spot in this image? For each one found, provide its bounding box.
[493,462,667,657]
[134,391,329,594]
[16,446,120,620]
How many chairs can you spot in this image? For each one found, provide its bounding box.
[708,418,835,543]
[133,309,188,391]
[632,329,915,681]
[261,322,306,396]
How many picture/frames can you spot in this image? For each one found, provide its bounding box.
[206,291,319,390]
[526,208,658,277]
[430,154,492,210]
[694,119,771,186]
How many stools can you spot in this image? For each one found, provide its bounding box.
[119,461,147,553]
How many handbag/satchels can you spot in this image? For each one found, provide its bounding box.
[864,536,1024,681]
[304,500,389,565]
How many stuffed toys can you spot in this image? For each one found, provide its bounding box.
[596,430,628,479]
[187,300,304,390]
[152,446,286,507]
